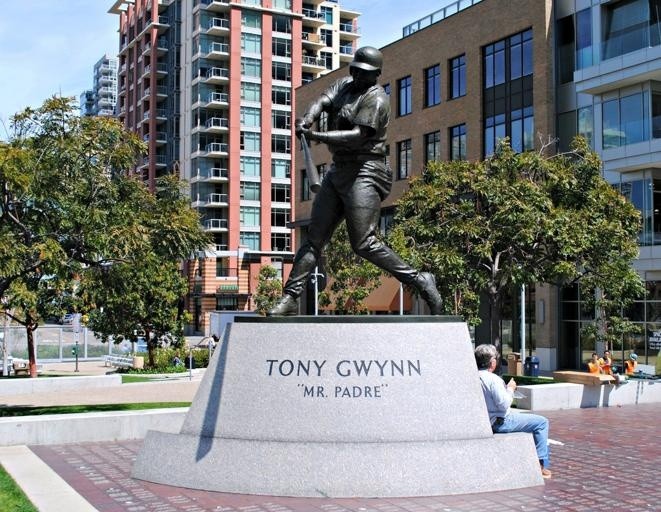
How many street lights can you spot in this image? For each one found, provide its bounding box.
[310,266,325,315]
[515,262,529,365]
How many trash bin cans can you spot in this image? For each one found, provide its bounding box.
[524,356,539,377]
[505,352,522,376]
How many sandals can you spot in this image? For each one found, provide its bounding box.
[541,467,552,479]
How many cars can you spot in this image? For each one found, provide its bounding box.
[137,336,149,354]
[64,314,73,324]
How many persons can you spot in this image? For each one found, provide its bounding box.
[173,351,184,368]
[587,353,603,375]
[475,343,554,478]
[185,352,196,368]
[624,353,639,375]
[600,351,615,376]
[269,47,444,315]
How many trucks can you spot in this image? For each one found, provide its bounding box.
[0,332,13,376]
[208,309,267,342]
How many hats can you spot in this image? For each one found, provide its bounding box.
[629,353,638,361]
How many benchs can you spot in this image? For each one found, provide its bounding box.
[13,361,44,376]
